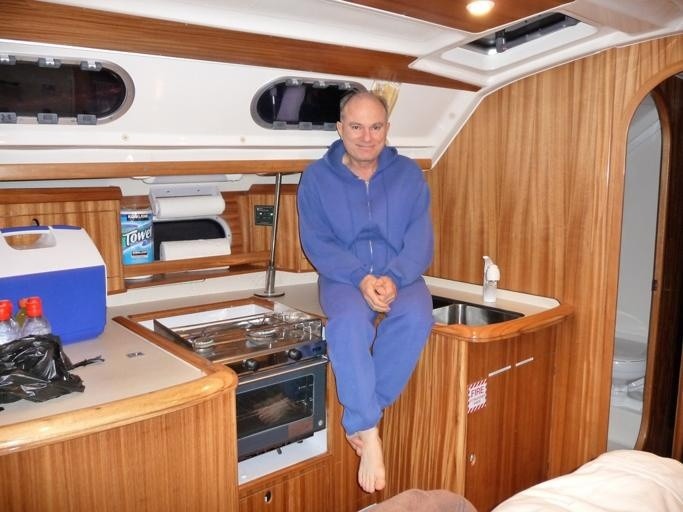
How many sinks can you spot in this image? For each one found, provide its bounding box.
[431,294,456,309]
[432,303,526,327]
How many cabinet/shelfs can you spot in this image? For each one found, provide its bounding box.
[464,325,558,507]
[236,457,337,510]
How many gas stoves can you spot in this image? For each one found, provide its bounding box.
[154,300,323,363]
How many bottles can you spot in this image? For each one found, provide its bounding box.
[0,297,51,347]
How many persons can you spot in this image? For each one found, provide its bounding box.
[292,85,438,494]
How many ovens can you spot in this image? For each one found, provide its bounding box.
[221,339,329,462]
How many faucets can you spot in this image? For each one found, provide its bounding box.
[482,254,501,304]
